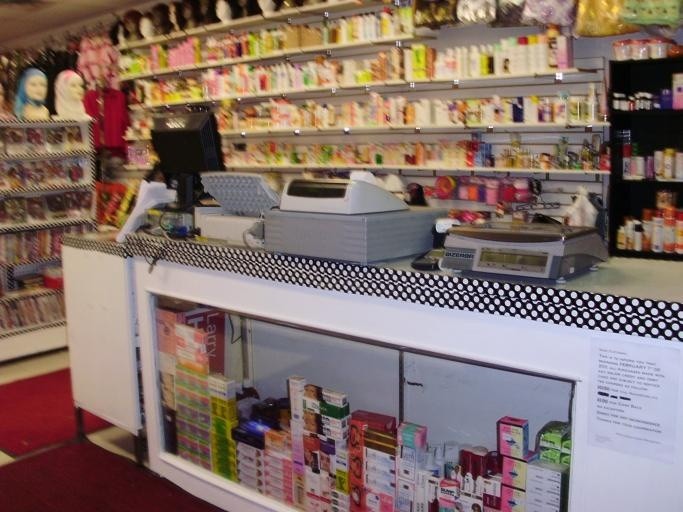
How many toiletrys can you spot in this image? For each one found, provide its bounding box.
[118,8,683,255]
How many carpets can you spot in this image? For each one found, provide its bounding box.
[0,440,231,511]
[1,367,117,456]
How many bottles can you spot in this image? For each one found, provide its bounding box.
[609,89,651,112]
[621,140,682,182]
[431,441,444,480]
[611,38,669,61]
[611,188,681,255]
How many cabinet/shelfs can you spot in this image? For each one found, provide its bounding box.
[110,1,683,262]
[56,232,144,470]
[0,107,98,366]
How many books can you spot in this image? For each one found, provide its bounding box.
[0,126,94,331]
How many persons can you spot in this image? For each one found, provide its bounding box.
[298,384,364,506]
[109,1,279,45]
[77,38,121,92]
[51,70,94,122]
[15,68,51,122]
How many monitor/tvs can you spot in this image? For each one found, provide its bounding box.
[149,111,227,213]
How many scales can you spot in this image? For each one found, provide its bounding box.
[438,224,609,285]
